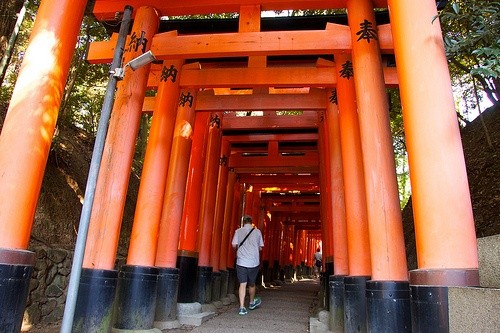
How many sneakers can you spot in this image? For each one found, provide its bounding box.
[249,296,261,309]
[238,307,248,316]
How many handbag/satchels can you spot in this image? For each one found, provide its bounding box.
[234,258,237,268]
[316,260,321,267]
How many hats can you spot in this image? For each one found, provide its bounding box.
[243,216,252,221]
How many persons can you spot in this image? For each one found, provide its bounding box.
[313,247,322,280]
[231,214,265,315]
